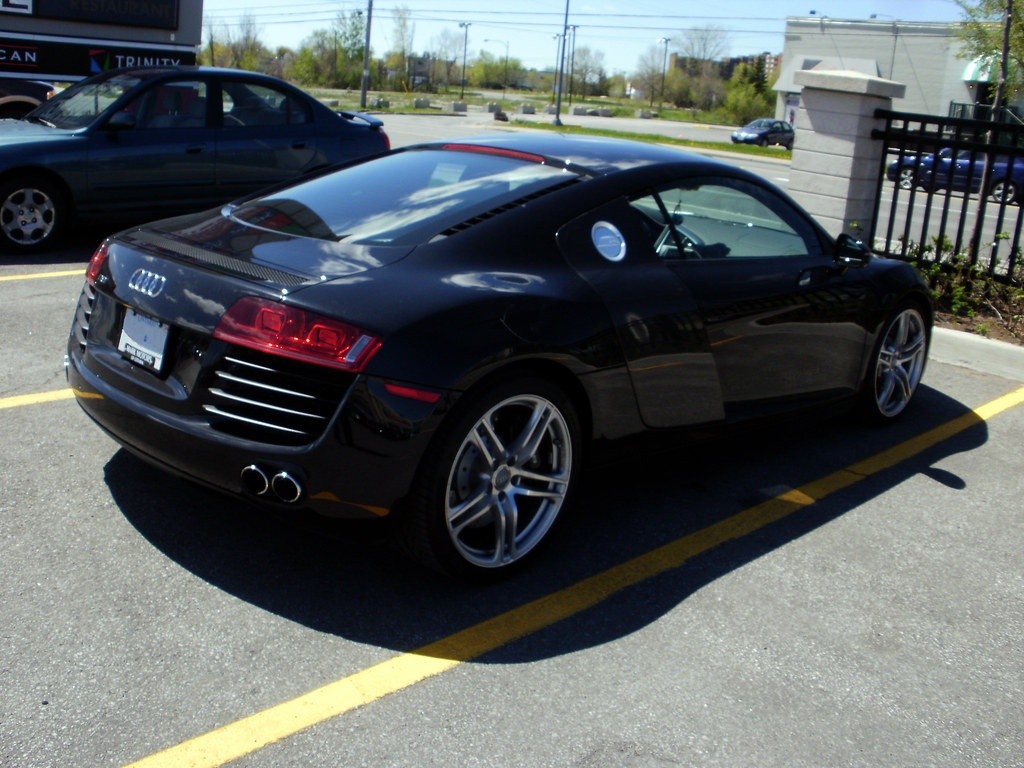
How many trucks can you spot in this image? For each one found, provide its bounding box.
[0,0,204,120]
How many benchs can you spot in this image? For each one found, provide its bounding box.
[231,106,305,125]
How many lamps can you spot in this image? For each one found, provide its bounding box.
[870,13,891,19]
[809,9,823,18]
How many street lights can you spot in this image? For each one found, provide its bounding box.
[551,25,579,106]
[460,23,471,98]
[659,38,671,111]
[484,39,509,100]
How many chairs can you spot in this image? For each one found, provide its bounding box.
[171,96,207,128]
[110,98,143,127]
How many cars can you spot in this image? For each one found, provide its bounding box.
[63,132,936,592]
[887,148,1024,205]
[731,119,794,150]
[0,65,390,258]
